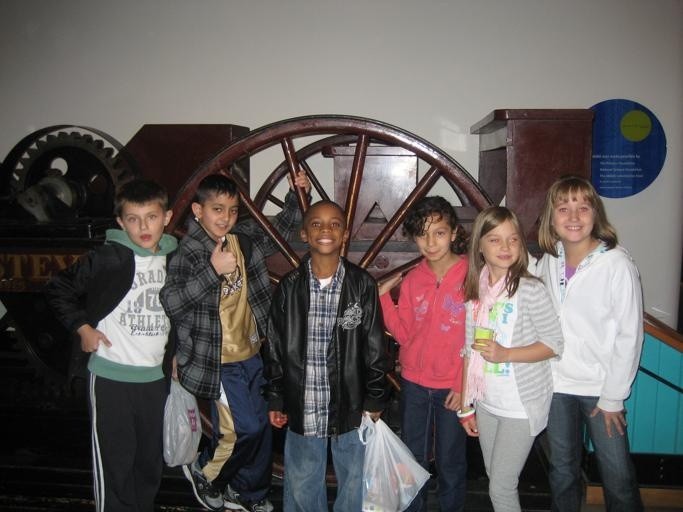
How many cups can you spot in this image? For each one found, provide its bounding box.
[474,326,493,352]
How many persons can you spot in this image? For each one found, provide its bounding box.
[44,177,178,511]
[159,168,312,512]
[378,196,470,512]
[263,200,433,512]
[456,206,564,512]
[535,176,644,512]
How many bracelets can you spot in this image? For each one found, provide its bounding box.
[458,408,476,423]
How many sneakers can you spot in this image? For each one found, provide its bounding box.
[220,483,274,512]
[181,450,225,511]
